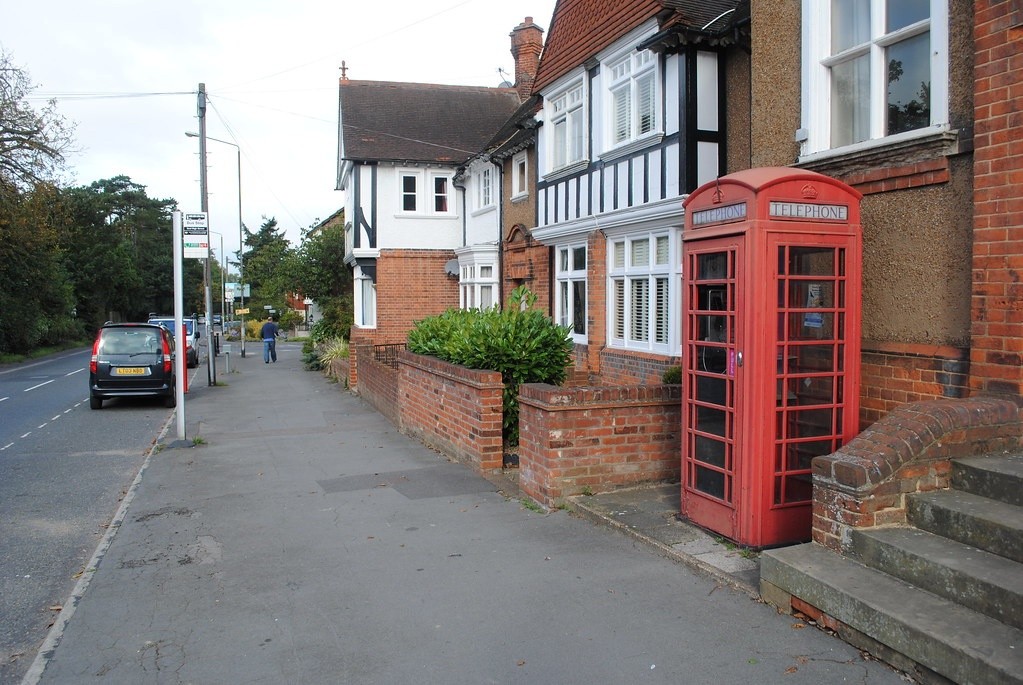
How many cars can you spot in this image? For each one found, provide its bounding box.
[89,320,176,409]
[197,316,205,324]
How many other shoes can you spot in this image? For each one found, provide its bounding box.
[265,362,269,364]
[273,359,277,363]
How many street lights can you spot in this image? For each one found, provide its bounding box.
[185,131,246,359]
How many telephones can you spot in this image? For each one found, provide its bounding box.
[712,296,724,328]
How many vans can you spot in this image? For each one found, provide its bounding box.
[148,317,200,369]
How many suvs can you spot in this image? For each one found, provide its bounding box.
[213,315,222,325]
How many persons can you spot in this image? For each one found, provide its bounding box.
[261,317,279,363]
